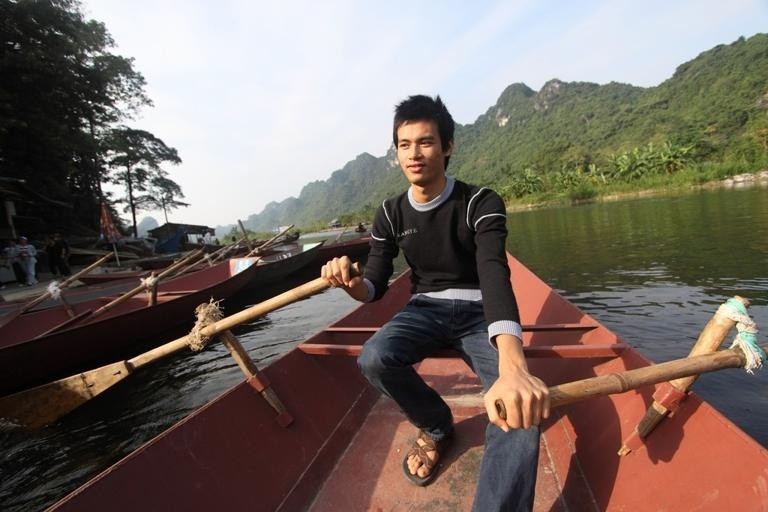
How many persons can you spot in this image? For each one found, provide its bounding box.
[15,236,39,287]
[3,238,28,287]
[321,94,553,511]
[45,236,61,277]
[52,230,72,278]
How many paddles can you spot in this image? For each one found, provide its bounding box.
[39,257,191,336]
[0,251,115,329]
[1,261,363,433]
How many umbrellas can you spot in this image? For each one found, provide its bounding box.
[99,201,124,266]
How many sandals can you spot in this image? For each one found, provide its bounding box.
[403,429,445,487]
